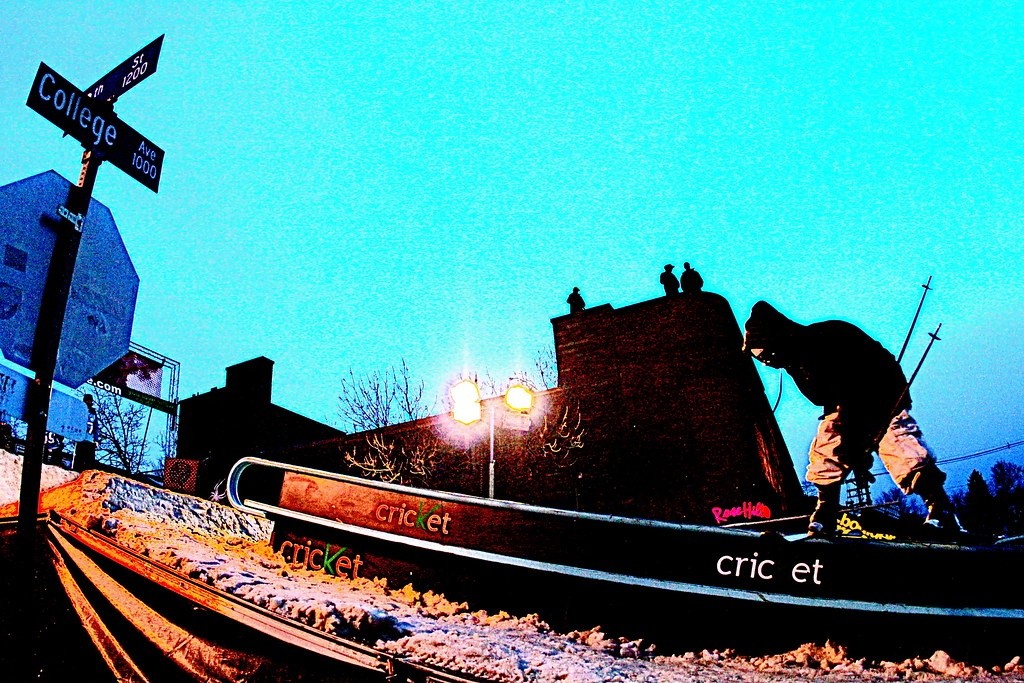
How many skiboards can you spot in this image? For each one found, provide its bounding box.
[860,510,998,544]
[764,524,865,543]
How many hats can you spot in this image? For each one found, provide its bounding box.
[573,287,580,291]
[739,330,764,351]
[664,264,675,269]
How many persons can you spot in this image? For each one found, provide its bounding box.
[70,394,99,473]
[745,303,977,545]
[660,264,680,296]
[568,286,585,314]
[680,262,703,292]
